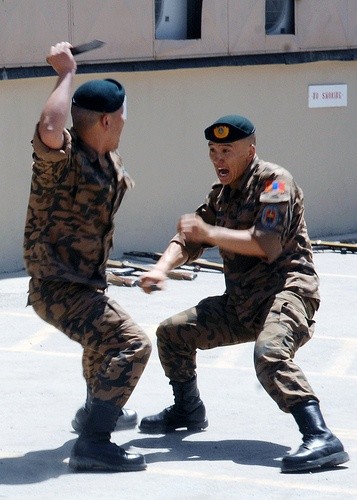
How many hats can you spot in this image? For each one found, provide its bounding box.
[71,78,125,113]
[202,115,255,143]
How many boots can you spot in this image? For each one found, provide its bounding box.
[68,401,146,471]
[280,399,349,471]
[70,386,138,431]
[138,378,208,434]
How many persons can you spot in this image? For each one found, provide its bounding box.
[138,115,350,472]
[24,42,152,470]
